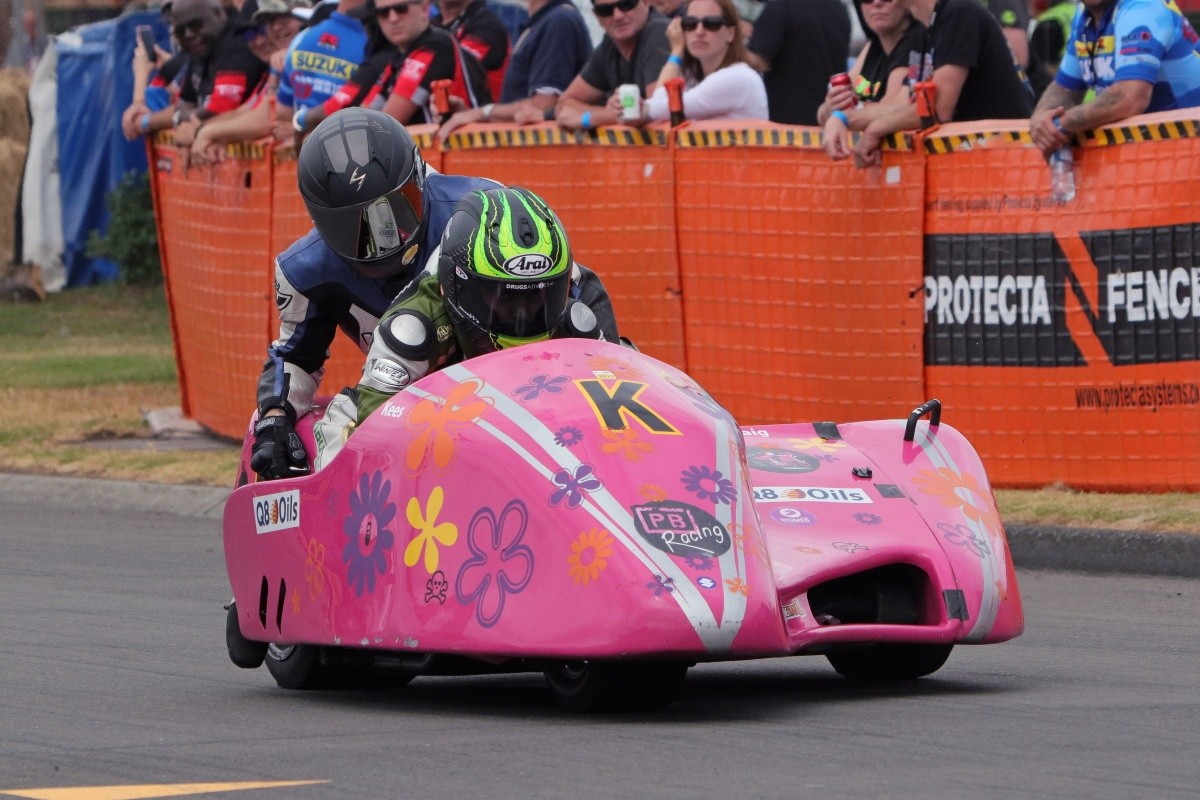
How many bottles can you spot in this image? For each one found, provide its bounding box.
[1049,145,1075,201]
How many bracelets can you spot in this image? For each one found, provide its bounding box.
[831,109,849,128]
[484,103,496,121]
[194,123,204,139]
[666,54,684,68]
[544,106,555,123]
[141,112,151,133]
[292,110,305,133]
[580,112,593,132]
[298,107,310,129]
[1054,114,1076,136]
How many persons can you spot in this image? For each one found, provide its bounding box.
[123,0,1200,177]
[313,185,620,477]
[251,108,509,481]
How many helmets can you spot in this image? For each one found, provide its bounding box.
[437,186,572,358]
[297,106,427,279]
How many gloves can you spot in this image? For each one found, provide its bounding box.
[249,416,311,480]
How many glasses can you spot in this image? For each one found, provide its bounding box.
[373,1,421,19]
[681,15,729,32]
[168,8,215,38]
[593,0,639,16]
[244,29,266,44]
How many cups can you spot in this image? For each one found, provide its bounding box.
[619,83,640,119]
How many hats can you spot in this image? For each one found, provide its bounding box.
[233,10,263,35]
[252,0,314,23]
[290,0,341,25]
